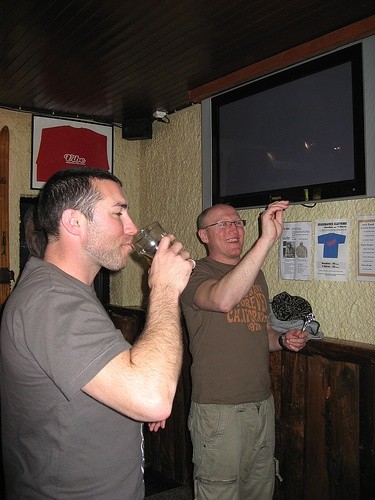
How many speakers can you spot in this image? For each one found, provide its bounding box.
[121,120,152,141]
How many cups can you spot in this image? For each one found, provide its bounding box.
[131,221,195,279]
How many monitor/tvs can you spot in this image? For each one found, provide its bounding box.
[210,41,366,210]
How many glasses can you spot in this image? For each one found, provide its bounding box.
[202,219,246,230]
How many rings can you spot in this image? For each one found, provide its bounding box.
[189,259,196,269]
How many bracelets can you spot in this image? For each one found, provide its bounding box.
[279,332,291,352]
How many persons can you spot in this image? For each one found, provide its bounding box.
[0,167,196,500]
[179,200,310,500]
